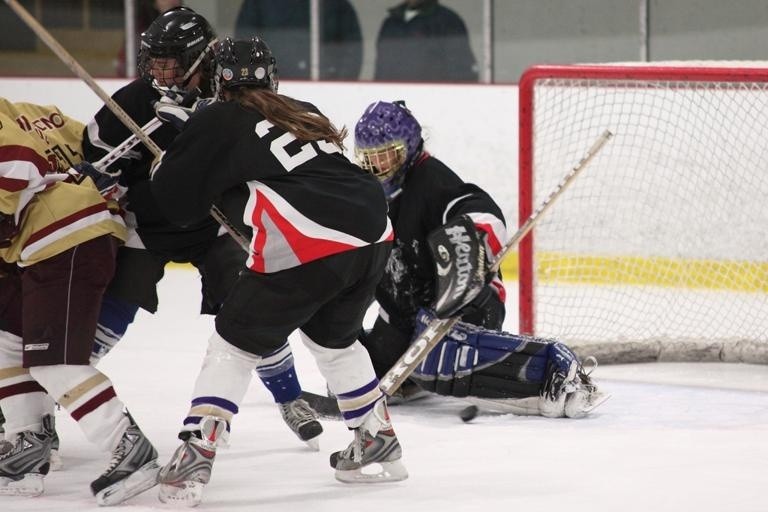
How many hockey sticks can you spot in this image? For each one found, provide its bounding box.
[297,132,612,425]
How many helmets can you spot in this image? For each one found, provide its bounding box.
[353,99,425,202]
[207,36,279,93]
[138,5,217,74]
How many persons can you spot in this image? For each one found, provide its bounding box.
[79,6,323,441]
[234,1,364,80]
[154,34,402,488]
[374,0,479,83]
[0,96,160,494]
[117,0,183,78]
[355,101,609,420]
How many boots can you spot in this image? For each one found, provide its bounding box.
[0,431,53,479]
[89,407,158,498]
[277,393,324,442]
[39,414,61,451]
[155,414,227,485]
[564,370,601,420]
[330,393,404,472]
[387,377,430,397]
